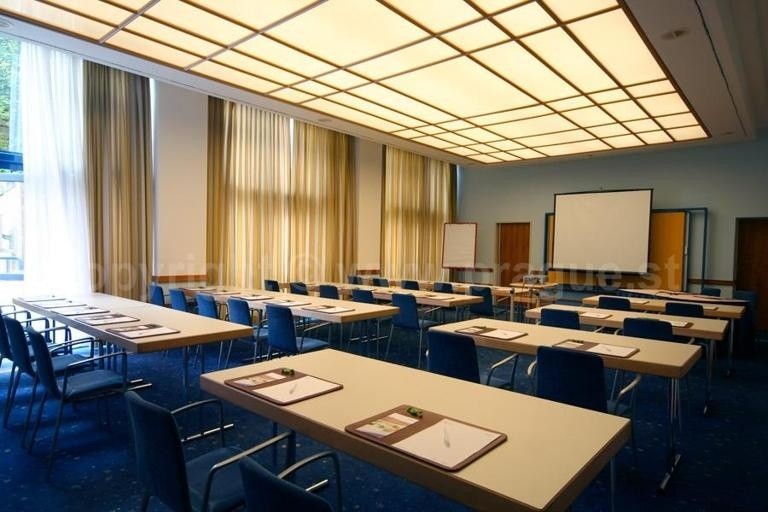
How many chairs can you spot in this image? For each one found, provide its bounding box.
[528,345,642,512]
[148,284,333,370]
[2,316,95,448]
[24,325,153,458]
[125,383,296,512]
[238,452,344,512]
[1,305,49,364]
[426,328,519,391]
[264,276,495,370]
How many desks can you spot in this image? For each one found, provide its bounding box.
[178,283,399,353]
[200,349,633,512]
[429,317,702,489]
[13,290,255,390]
[525,287,746,384]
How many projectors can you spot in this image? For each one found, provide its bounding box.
[522,275,548,284]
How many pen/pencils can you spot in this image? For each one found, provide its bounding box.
[442,426,449,447]
[602,346,611,351]
[290,381,296,395]
